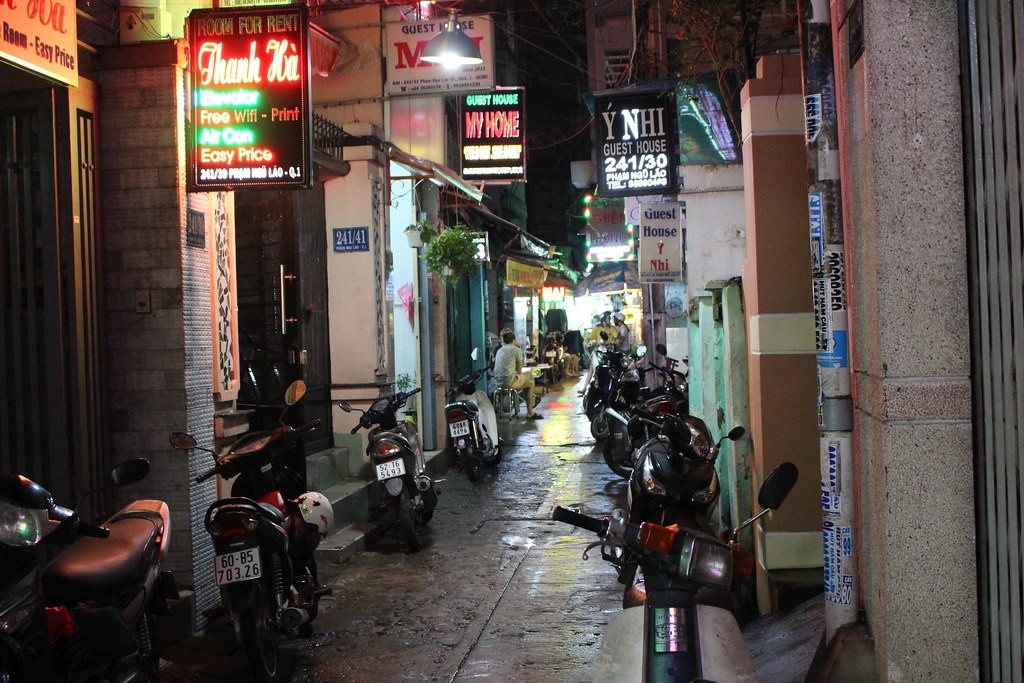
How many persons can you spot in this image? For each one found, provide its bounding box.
[492,327,514,376]
[493,332,544,419]
[613,312,630,353]
[577,309,623,395]
[547,332,582,376]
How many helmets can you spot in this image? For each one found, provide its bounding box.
[289,493,335,534]
[613,313,625,322]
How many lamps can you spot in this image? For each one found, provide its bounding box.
[420,7,484,65]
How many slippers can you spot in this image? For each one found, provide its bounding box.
[526,413,545,419]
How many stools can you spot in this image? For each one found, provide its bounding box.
[493,387,519,421]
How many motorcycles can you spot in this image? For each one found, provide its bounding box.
[444,365,504,482]
[578,330,689,478]
[544,330,565,384]
[0,458,179,683]
[551,462,799,683]
[167,380,335,683]
[626,403,746,531]
[339,388,446,553]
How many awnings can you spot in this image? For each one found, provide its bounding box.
[388,148,482,206]
[469,203,549,253]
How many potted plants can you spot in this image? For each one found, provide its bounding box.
[402,220,438,248]
[421,222,483,289]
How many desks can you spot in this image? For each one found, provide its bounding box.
[523,364,554,394]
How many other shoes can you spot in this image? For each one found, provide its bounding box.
[571,372,577,376]
[565,372,572,376]
